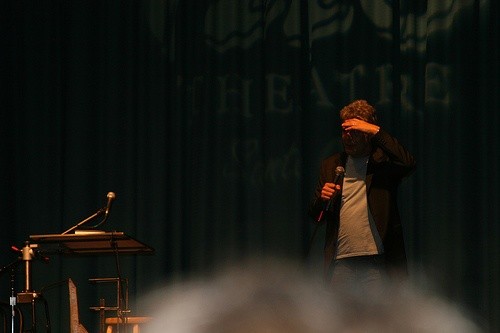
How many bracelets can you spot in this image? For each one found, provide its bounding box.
[375,127,380,135]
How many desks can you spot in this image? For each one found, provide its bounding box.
[29,232,155,333]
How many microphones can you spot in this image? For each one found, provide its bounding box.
[323,165,345,213]
[105,191,116,217]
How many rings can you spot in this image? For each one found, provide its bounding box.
[353,122,356,125]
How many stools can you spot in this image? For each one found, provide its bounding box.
[106,317,145,333]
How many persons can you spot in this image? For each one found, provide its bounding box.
[308,100,416,279]
